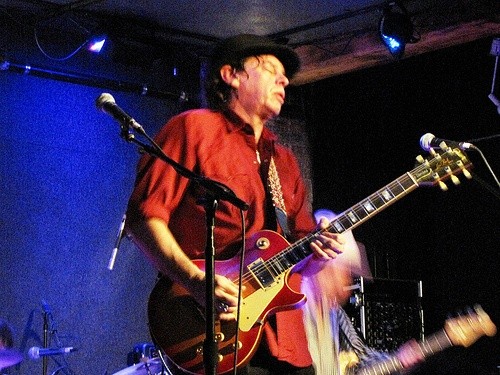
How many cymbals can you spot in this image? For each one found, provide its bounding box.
[112,356,163,375]
[0,347,25,369]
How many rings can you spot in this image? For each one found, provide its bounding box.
[216,302,228,312]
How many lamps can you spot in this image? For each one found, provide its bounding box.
[74,21,108,55]
[379,6,420,55]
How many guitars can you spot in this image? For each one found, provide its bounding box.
[147,140,474,375]
[337,305,496,375]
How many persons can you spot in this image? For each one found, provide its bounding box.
[127,33,345,375]
[299,209,425,375]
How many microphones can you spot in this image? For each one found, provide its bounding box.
[417,134,477,152]
[27,347,78,359]
[107,214,127,271]
[96,92,146,134]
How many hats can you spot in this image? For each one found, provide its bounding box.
[315,210,362,272]
[207,34,303,84]
